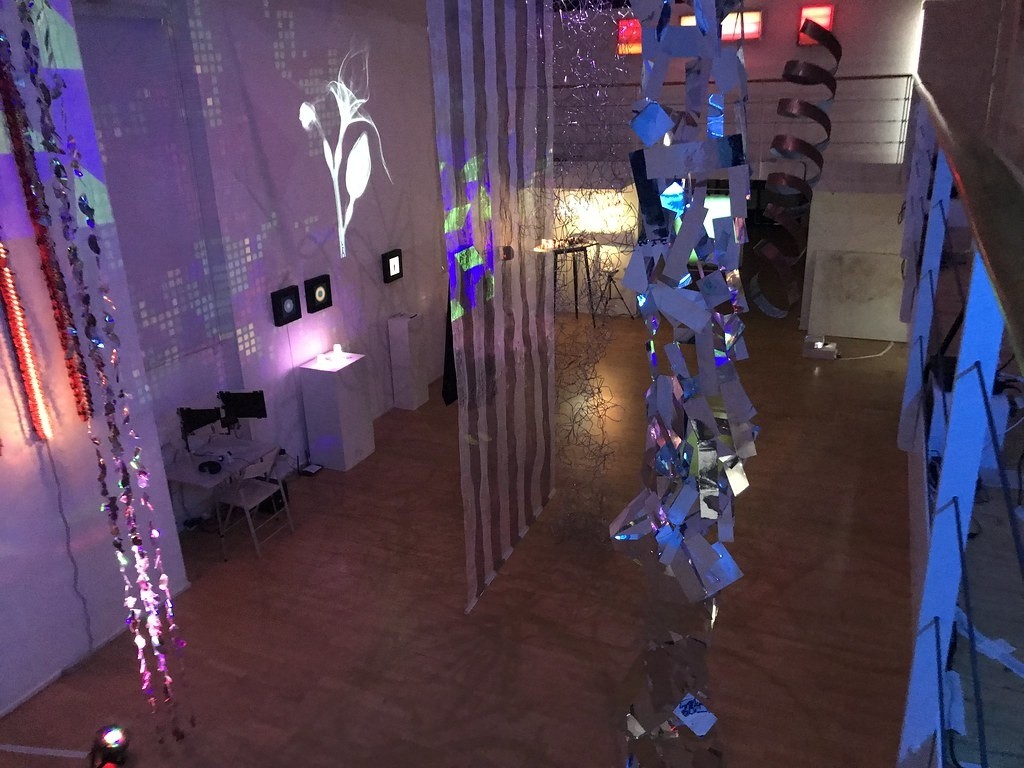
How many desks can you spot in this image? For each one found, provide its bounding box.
[165,436,278,561]
[533,240,600,329]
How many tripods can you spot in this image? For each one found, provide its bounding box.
[595,274,635,326]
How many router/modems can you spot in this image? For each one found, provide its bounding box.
[297,450,324,476]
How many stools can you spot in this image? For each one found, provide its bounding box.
[213,470,295,559]
